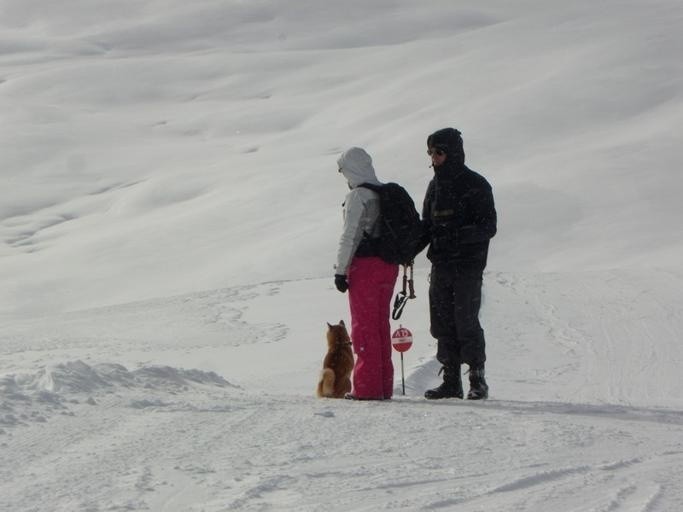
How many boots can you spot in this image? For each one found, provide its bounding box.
[468,365,489,400]
[425,365,464,401]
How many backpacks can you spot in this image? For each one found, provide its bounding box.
[356,182,431,265]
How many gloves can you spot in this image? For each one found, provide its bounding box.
[334,274,349,293]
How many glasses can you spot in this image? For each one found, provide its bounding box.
[427,148,444,155]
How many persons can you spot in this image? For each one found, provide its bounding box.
[333,144,402,400]
[419,127,498,400]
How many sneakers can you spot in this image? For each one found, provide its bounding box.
[345,392,392,401]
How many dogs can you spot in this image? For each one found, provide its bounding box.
[316,319,354,398]
